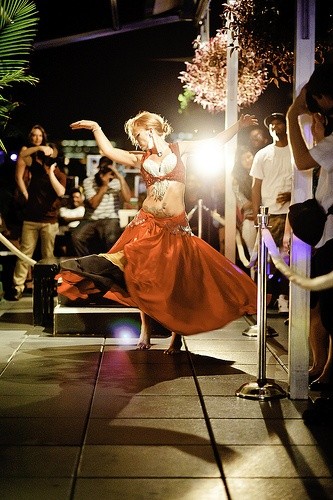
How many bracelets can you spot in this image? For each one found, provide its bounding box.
[92,127,102,132]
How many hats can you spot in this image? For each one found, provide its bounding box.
[264,113,286,128]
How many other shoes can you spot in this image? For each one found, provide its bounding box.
[11,289,22,301]
[283,318,289,324]
[308,378,333,392]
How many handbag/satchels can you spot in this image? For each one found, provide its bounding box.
[288,198,327,246]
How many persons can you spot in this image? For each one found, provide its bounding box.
[10,64,333,425]
[54,111,272,356]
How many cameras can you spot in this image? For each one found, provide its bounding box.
[102,168,110,174]
[37,151,54,167]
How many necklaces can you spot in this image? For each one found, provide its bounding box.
[156,151,163,157]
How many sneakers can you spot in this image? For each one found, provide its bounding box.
[277,294,289,314]
